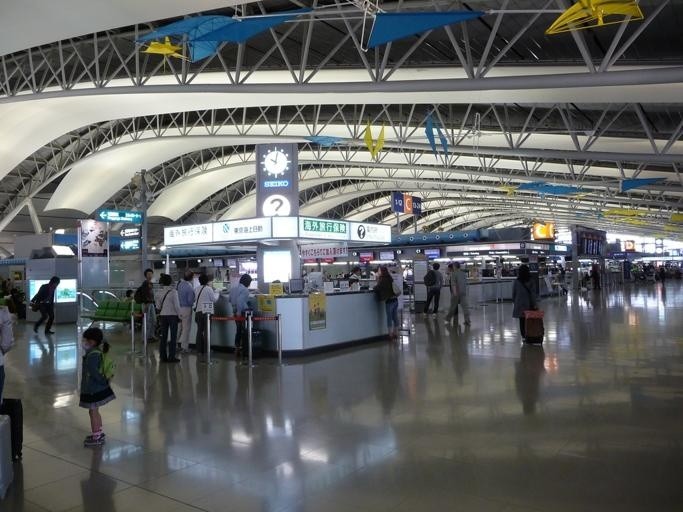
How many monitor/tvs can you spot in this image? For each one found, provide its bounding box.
[379,250,395,260]
[424,249,440,260]
[359,250,374,261]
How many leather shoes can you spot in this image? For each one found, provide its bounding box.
[385,335,397,340]
[33,324,37,333]
[44,330,55,335]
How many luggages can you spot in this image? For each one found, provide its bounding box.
[523,310,545,346]
[520,345,545,375]
[0,461,23,512]
[0,397,23,461]
[241,308,265,360]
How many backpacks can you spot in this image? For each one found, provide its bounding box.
[133,280,147,304]
[86,348,116,380]
[28,292,39,312]
[423,269,436,286]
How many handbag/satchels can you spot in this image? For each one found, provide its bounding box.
[391,280,402,296]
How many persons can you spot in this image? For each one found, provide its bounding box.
[0,303,14,410]
[124,267,259,364]
[512,268,540,342]
[132,366,264,450]
[30,276,59,334]
[78,446,116,512]
[76,327,116,447]
[517,346,543,415]
[372,321,472,417]
[336,260,470,342]
[32,334,62,389]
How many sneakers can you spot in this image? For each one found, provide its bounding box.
[82,432,106,448]
[197,349,213,356]
[141,335,159,344]
[179,347,192,354]
[159,357,180,363]
